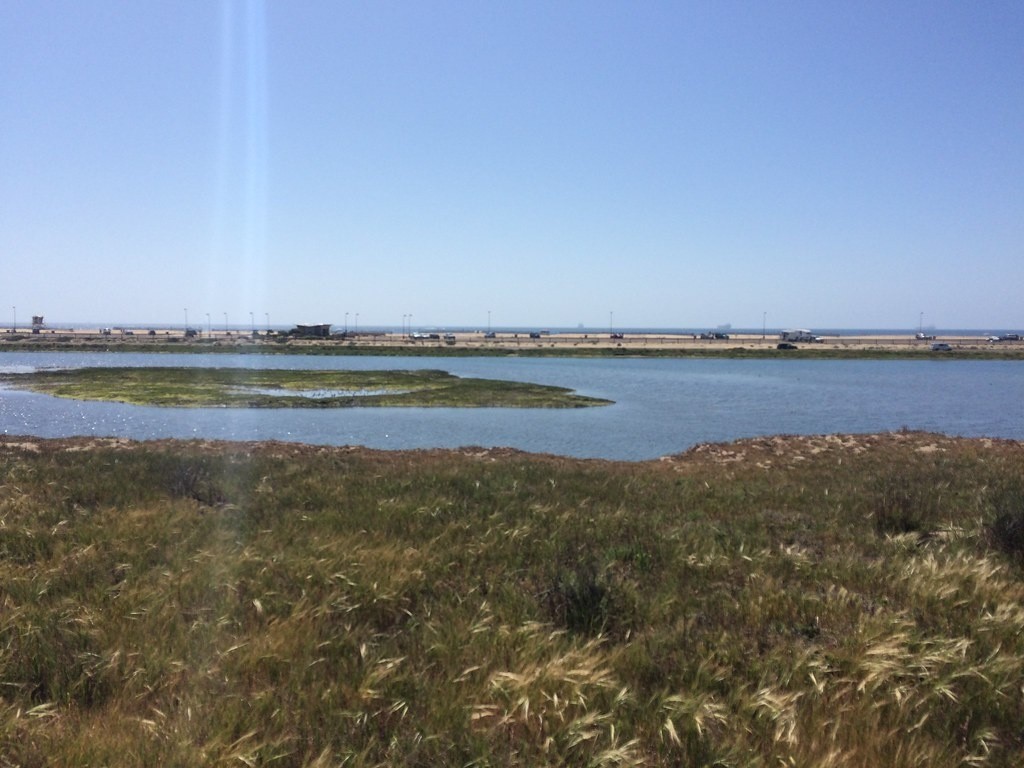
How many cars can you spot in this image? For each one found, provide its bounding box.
[149,330,155,335]
[411,332,441,340]
[985,334,1018,342]
[125,331,133,335]
[777,343,797,350]
[485,332,495,338]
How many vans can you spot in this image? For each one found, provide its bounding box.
[928,343,952,352]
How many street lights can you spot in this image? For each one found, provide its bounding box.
[355,312,359,334]
[402,313,413,339]
[12,305,17,333]
[344,311,349,334]
[249,311,254,333]
[183,308,188,330]
[205,313,211,335]
[223,312,228,333]
[265,312,271,330]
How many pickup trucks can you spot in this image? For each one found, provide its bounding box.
[442,332,456,340]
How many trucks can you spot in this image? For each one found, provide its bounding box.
[530,331,540,338]
[780,328,816,343]
[699,332,730,339]
